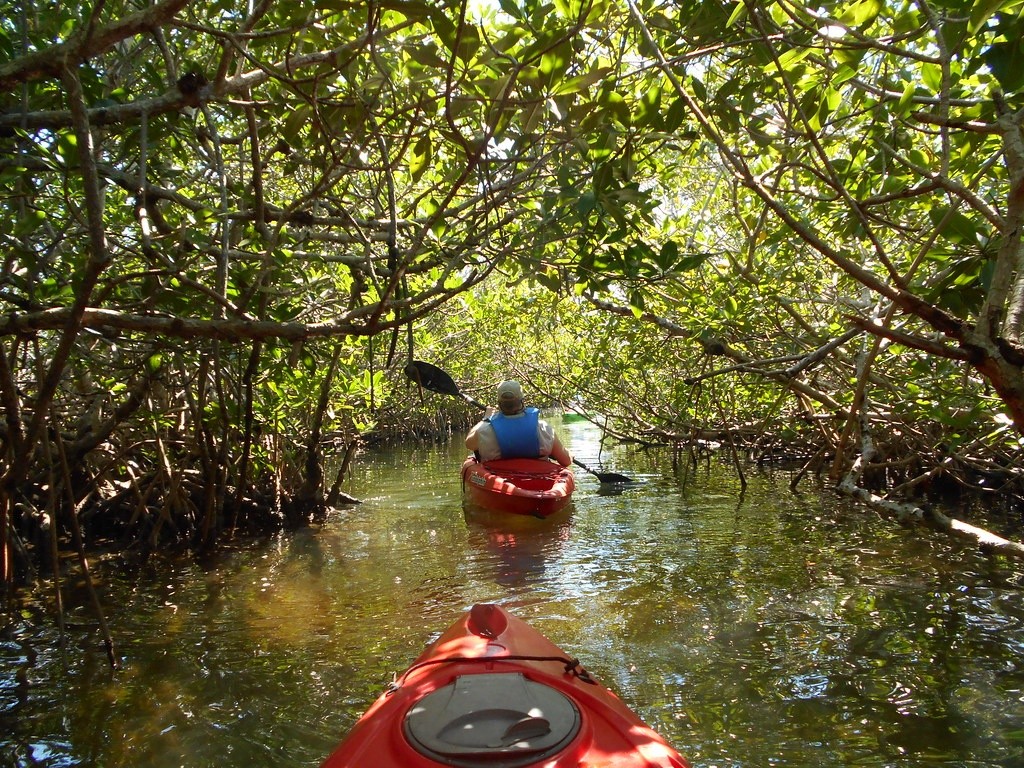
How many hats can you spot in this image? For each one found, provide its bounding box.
[497,380,523,402]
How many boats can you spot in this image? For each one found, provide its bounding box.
[561,410,594,421]
[459,452,574,520]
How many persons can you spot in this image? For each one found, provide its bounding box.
[466,381,572,468]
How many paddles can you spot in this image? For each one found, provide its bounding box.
[402,359,634,485]
